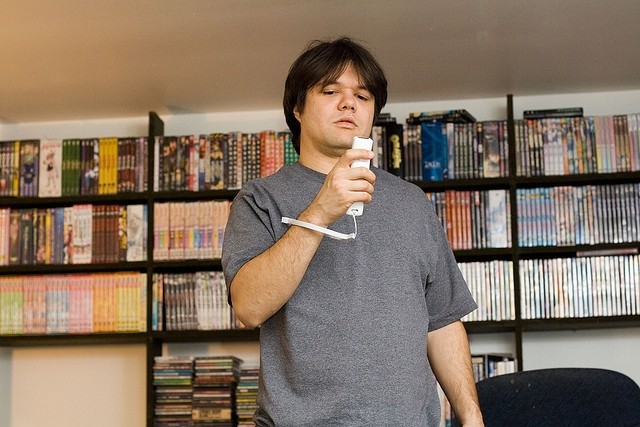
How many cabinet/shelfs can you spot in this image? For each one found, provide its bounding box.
[0,108,636,426]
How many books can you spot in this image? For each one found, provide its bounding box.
[440,352,517,426]
[153,131,296,190]
[152,273,246,333]
[517,180,639,254]
[424,188,513,251]
[516,252,640,335]
[1,272,149,334]
[1,137,147,192]
[370,108,507,180]
[513,117,638,200]
[146,357,258,426]
[1,206,147,260]
[450,255,512,322]
[152,203,231,262]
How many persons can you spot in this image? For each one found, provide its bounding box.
[220,36,487,427]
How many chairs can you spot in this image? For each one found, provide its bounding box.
[459,363,637,426]
